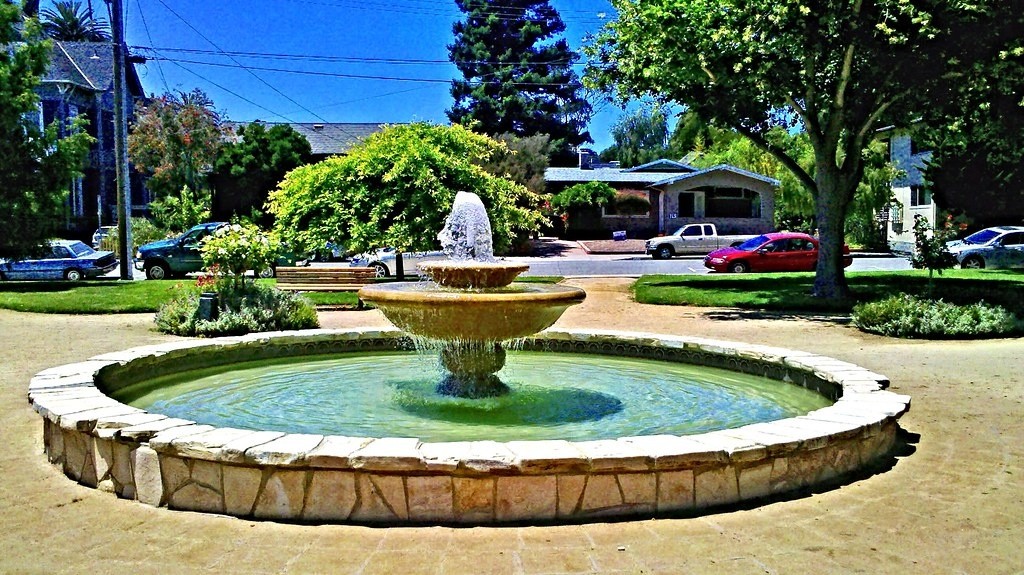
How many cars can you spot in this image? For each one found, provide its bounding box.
[702,232,853,273]
[0,240,120,281]
[349,246,445,278]
[282,240,346,262]
[937,226,1024,270]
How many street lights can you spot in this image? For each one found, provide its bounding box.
[90,44,134,280]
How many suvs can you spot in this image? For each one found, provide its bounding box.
[92,226,118,250]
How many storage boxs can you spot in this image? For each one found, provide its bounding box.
[613,231,626,240]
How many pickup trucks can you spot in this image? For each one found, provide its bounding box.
[645,223,761,260]
[133,222,316,278]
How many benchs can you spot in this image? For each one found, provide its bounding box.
[276,267,376,308]
